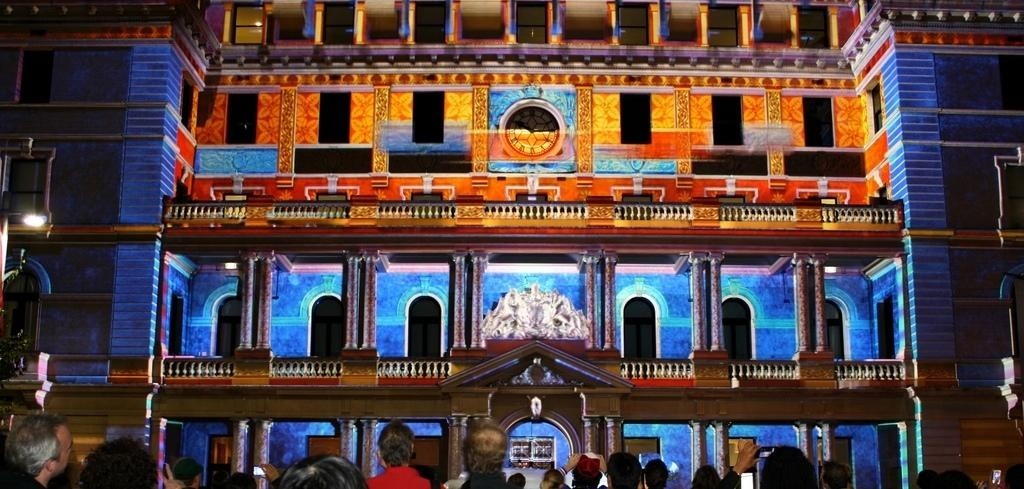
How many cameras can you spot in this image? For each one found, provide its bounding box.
[757,446,774,460]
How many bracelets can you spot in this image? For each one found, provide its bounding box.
[732,467,742,477]
[604,471,608,478]
[559,467,566,476]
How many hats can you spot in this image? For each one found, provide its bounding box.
[173,458,203,480]
[576,455,600,478]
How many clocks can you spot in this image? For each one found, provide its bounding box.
[498,98,566,162]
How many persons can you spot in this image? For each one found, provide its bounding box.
[606,451,643,489]
[443,469,473,489]
[461,417,510,488]
[718,436,821,488]
[79,436,185,489]
[215,461,282,489]
[363,419,433,489]
[643,457,672,489]
[821,457,853,488]
[1,412,76,488]
[274,454,366,489]
[538,450,611,489]
[691,464,722,488]
[173,455,205,489]
